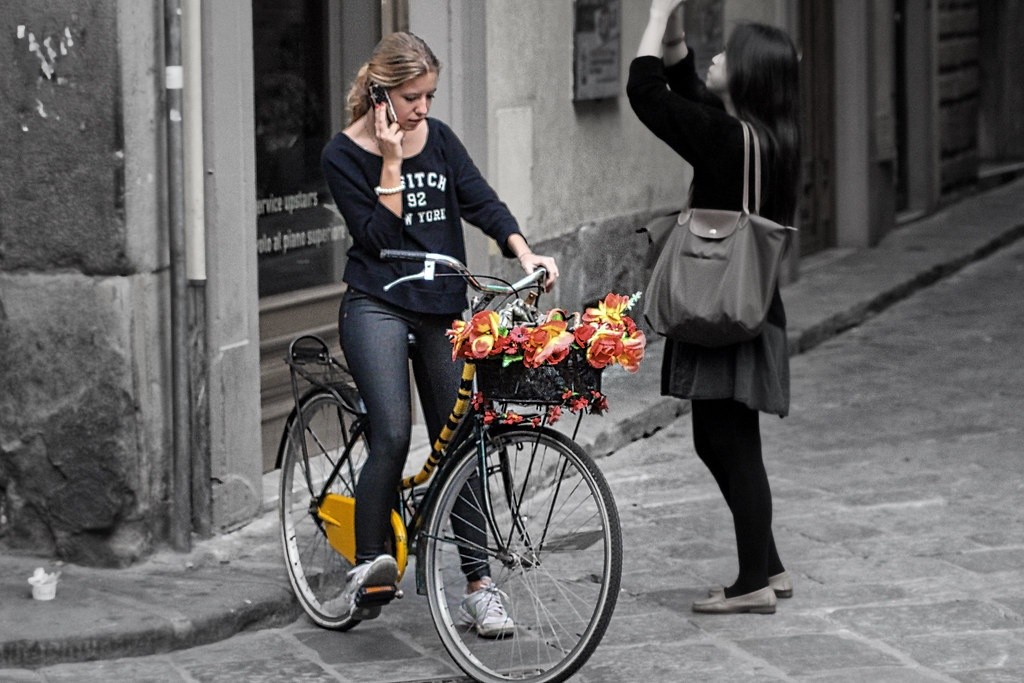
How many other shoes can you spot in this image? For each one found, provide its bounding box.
[709,571,792,598]
[692,587,777,613]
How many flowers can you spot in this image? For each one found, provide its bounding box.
[445,291,647,428]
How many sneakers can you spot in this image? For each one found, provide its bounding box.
[458,576,514,637]
[347,554,397,620]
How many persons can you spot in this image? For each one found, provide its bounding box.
[625,0,800,615]
[320,31,561,640]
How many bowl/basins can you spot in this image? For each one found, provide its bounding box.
[28,576,56,600]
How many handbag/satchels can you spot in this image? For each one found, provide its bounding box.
[638,118,797,346]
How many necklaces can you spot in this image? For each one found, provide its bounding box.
[362,116,407,149]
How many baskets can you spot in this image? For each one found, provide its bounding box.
[469,330,603,404]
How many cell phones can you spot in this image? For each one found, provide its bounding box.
[369,83,397,126]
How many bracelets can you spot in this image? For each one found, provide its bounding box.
[661,31,686,46]
[374,185,406,196]
[519,252,535,262]
